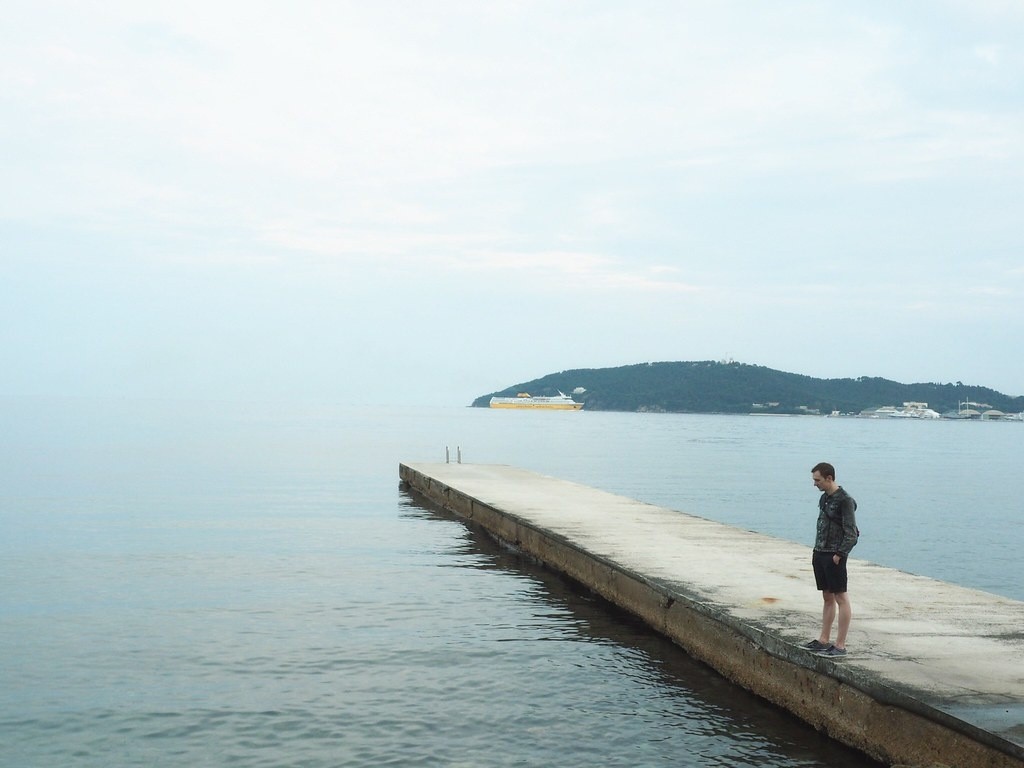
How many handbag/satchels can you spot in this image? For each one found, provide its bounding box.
[856,525,860,538]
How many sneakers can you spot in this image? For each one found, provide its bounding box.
[817,646,847,658]
[799,640,831,651]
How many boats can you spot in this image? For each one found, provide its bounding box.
[490,388,586,410]
[887,396,974,419]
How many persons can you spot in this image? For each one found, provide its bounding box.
[799,462,859,658]
